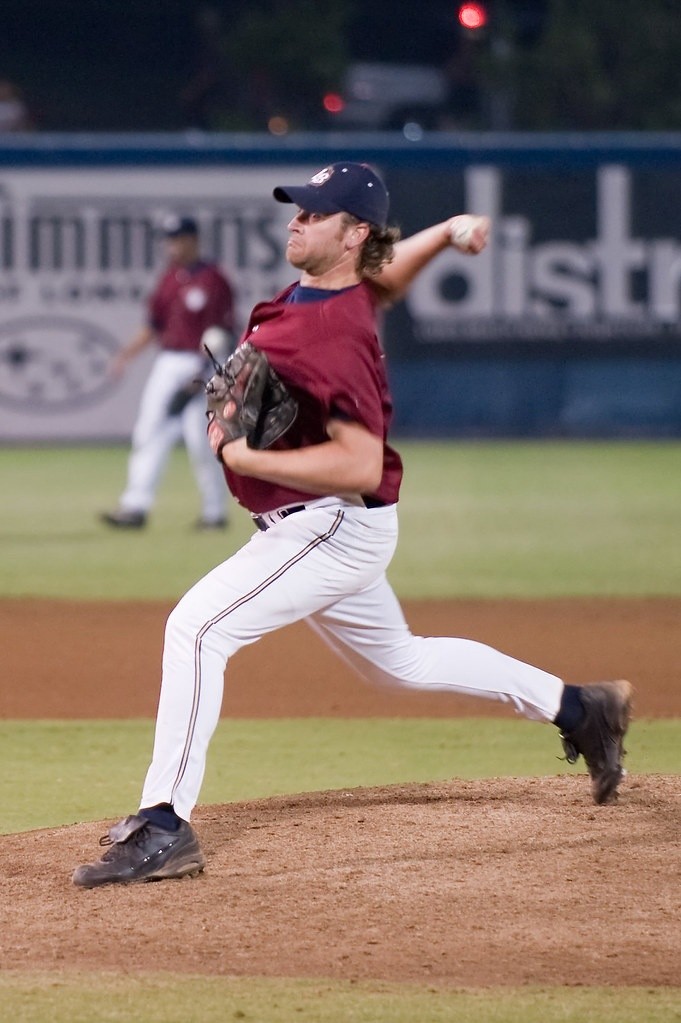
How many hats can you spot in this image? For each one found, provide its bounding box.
[154,218,196,236]
[273,161,389,228]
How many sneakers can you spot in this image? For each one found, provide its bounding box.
[556,681,636,806]
[72,802,206,888]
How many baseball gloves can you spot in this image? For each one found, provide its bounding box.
[204,340,299,463]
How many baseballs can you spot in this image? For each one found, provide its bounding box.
[450,214,482,246]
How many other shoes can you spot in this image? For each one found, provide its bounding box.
[104,508,143,527]
[197,514,225,528]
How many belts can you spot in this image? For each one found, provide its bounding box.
[251,493,387,531]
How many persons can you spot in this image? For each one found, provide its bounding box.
[72,161,634,888]
[101,215,239,531]
